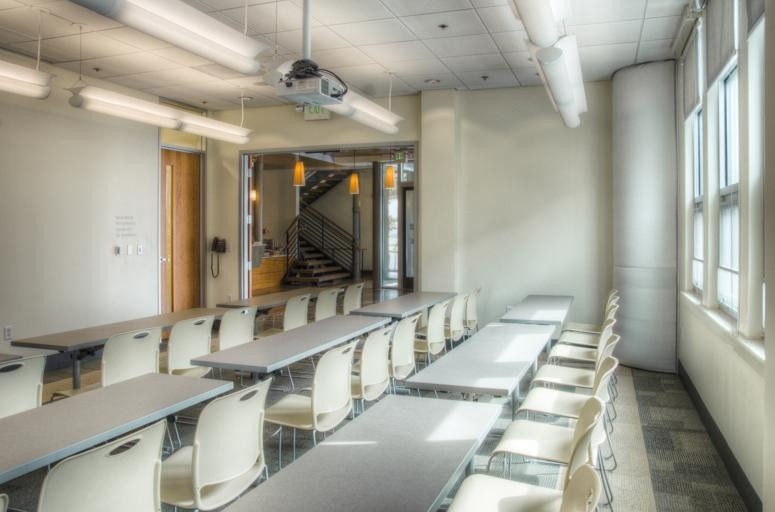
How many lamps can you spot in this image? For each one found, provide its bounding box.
[384,146,396,190]
[317,71,404,135]
[73,0,272,77]
[349,149,360,195]
[536,47,584,130]
[292,152,305,188]
[514,0,559,49]
[1,38,57,100]
[67,25,254,146]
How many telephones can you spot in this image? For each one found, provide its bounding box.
[210,236,226,256]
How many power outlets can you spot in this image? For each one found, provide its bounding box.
[4,325,12,340]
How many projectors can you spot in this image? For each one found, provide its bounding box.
[277,60,345,107]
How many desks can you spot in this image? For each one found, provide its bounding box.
[220,393,503,510]
[0,345,61,373]
[404,322,559,422]
[349,291,458,334]
[189,318,394,391]
[10,307,241,390]
[216,287,345,334]
[0,372,234,512]
[499,294,575,357]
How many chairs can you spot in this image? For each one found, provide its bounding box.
[254,293,316,391]
[38,419,168,512]
[417,296,465,351]
[446,464,601,512]
[160,314,215,451]
[414,303,449,399]
[445,289,479,338]
[352,313,420,396]
[51,326,175,454]
[515,356,618,474]
[543,318,617,392]
[213,305,258,377]
[263,338,360,470]
[558,288,620,348]
[487,395,615,508]
[336,282,365,315]
[308,287,344,322]
[351,324,392,417]
[524,334,619,419]
[159,378,273,512]
